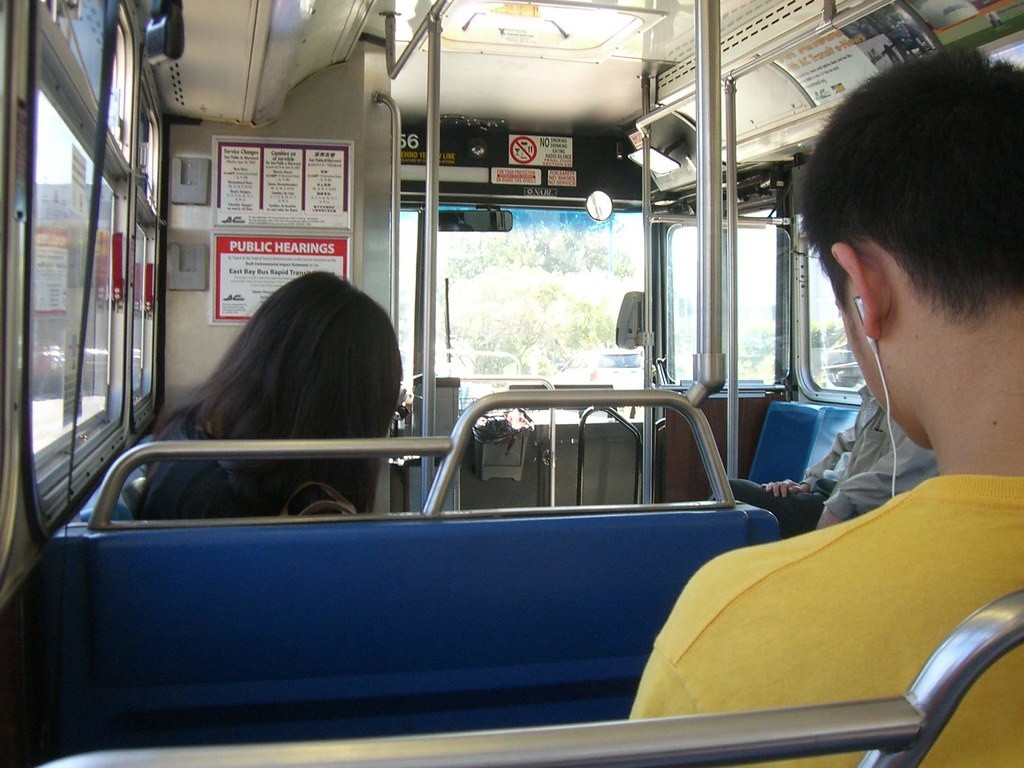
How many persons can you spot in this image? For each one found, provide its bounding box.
[713,385,940,540]
[628,46,1024,767]
[136,271,404,520]
[439,213,464,230]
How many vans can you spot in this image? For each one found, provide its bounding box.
[553,348,644,391]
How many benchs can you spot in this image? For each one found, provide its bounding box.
[15,390,1024,768]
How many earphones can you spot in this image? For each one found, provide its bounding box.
[853,296,879,355]
